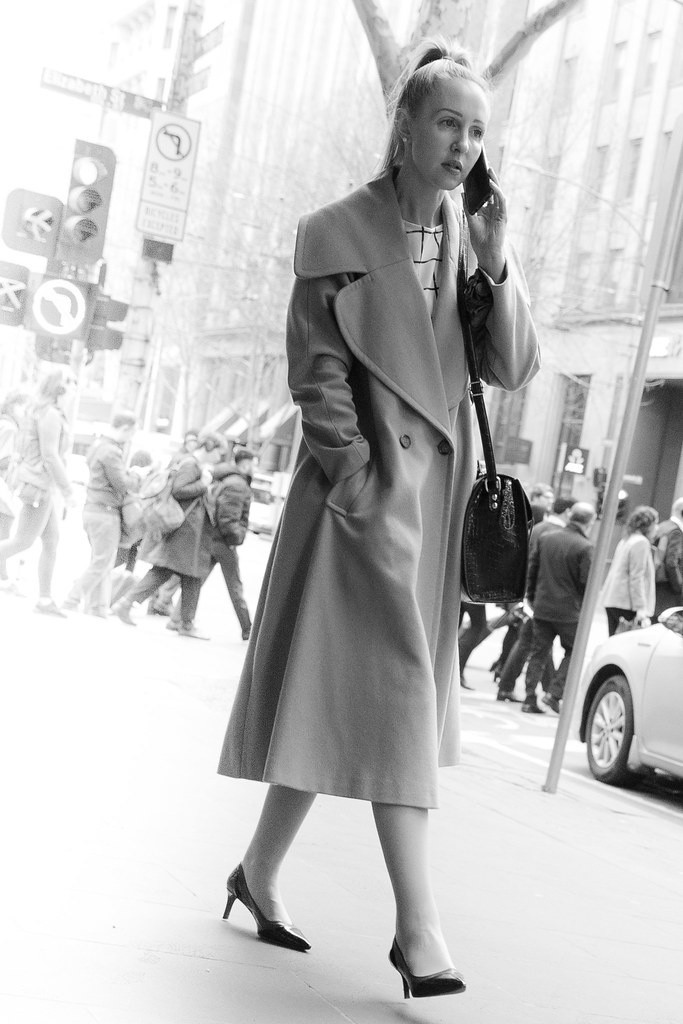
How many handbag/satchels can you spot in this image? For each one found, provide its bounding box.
[614,615,643,635]
[142,492,186,541]
[13,463,52,508]
[460,470,535,603]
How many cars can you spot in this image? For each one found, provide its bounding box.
[576,604,683,798]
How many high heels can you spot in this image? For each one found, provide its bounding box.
[223,864,312,951]
[460,674,473,691]
[388,937,466,1000]
[489,662,501,682]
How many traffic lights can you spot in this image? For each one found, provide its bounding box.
[83,263,130,362]
[0,139,116,286]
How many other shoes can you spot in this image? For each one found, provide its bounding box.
[92,605,109,619]
[542,696,560,713]
[110,596,137,626]
[61,599,79,612]
[166,620,181,631]
[153,600,174,617]
[521,703,546,714]
[178,625,210,640]
[497,688,523,702]
[31,599,68,619]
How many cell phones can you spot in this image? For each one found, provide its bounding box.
[462,151,495,217]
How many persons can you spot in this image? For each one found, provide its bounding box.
[1,371,260,645]
[217,40,549,999]
[455,484,683,715]
